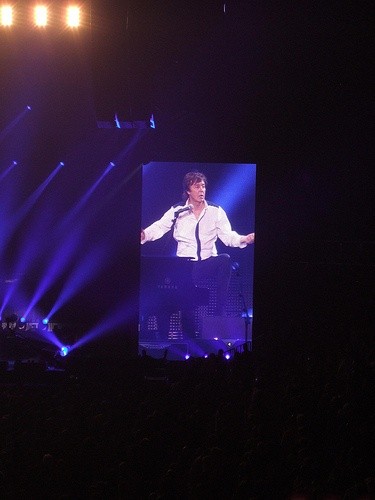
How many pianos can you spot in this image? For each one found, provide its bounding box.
[142,255,210,342]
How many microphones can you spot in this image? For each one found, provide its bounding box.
[177,204,194,213]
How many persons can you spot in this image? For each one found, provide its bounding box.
[141,172,254,338]
[1,345,375,500]
